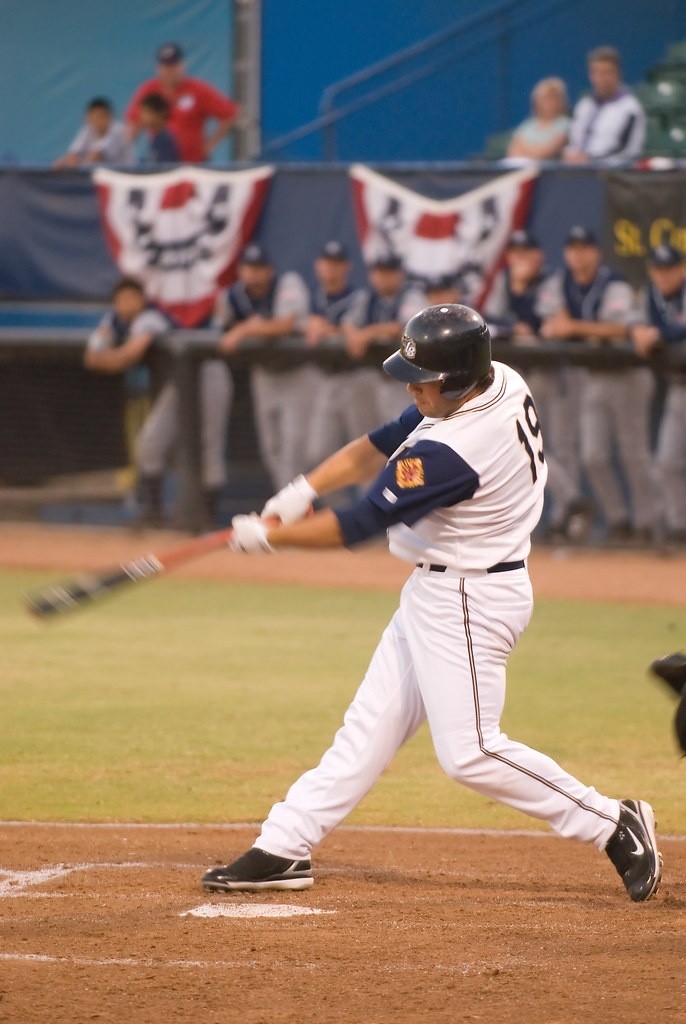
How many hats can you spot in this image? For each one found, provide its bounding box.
[504,232,540,251]
[649,245,681,268]
[563,226,599,247]
[240,244,271,268]
[156,45,183,65]
[432,271,461,291]
[364,246,404,270]
[313,241,354,261]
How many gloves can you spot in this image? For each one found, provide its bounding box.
[226,514,277,556]
[260,473,318,531]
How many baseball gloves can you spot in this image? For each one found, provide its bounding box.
[648,653,686,755]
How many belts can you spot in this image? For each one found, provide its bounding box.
[417,560,524,574]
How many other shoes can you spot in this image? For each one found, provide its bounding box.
[202,491,222,534]
[133,475,163,530]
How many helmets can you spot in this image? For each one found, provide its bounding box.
[383,303,492,396]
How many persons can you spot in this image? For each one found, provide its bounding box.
[124,43,238,162]
[211,244,311,484]
[485,229,595,544]
[632,244,686,543]
[505,76,571,160]
[534,225,657,541]
[347,251,511,424]
[53,97,138,165]
[200,303,663,901]
[141,94,180,161]
[563,46,646,167]
[294,242,381,509]
[83,276,183,526]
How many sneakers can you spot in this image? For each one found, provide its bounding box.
[605,798,663,903]
[199,848,315,894]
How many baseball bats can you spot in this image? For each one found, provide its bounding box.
[27,498,322,620]
